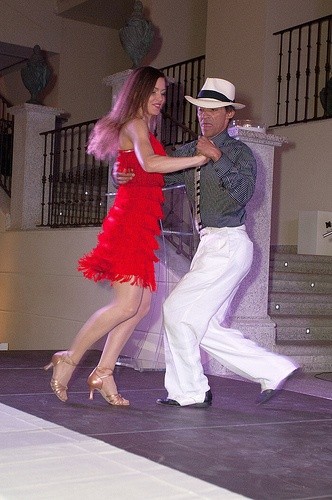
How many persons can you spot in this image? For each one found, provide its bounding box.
[43,65,216,406]
[109,77,299,409]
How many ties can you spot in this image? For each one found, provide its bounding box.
[194,166,203,233]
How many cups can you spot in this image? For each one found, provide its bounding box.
[229,119,264,129]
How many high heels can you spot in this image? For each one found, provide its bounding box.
[87,365,131,407]
[44,350,79,402]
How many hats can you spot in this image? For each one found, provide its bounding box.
[185,76,247,110]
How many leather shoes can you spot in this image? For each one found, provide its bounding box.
[156,390,213,409]
[254,367,300,406]
